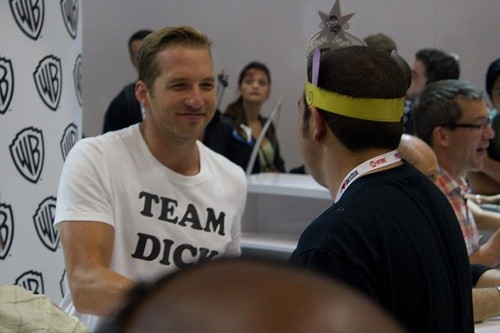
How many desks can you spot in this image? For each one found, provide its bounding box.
[241,172,333,261]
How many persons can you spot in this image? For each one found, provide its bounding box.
[286,45,475,333]
[54,25,248,333]
[90,254,405,333]
[102,29,500,333]
[404,80,500,268]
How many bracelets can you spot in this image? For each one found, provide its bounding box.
[495,286,500,292]
[475,194,481,204]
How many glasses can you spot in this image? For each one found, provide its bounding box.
[455,117,491,136]
